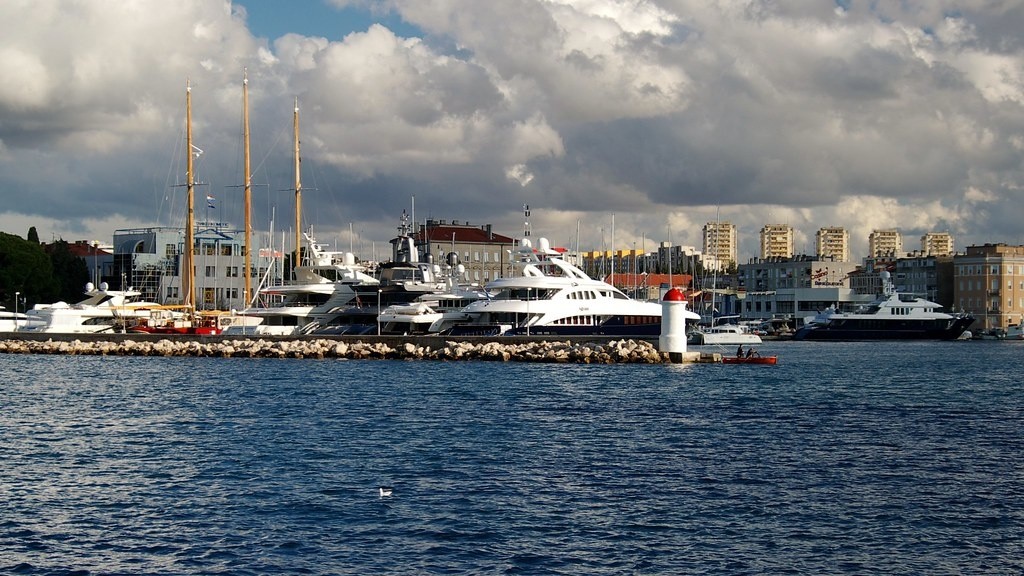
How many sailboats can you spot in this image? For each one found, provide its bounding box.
[377,230,491,324]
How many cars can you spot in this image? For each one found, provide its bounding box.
[753,327,767,336]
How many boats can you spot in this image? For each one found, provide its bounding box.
[0,303,51,336]
[23,282,170,334]
[788,270,975,341]
[220,226,493,338]
[978,323,1024,341]
[690,324,764,344]
[427,237,703,331]
